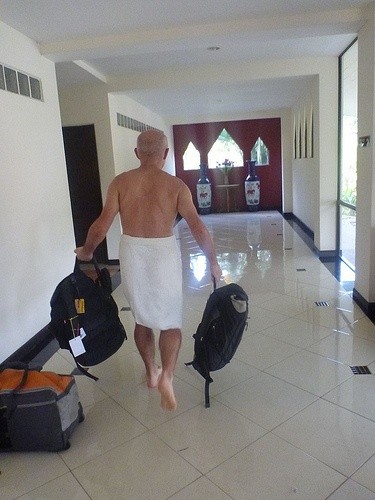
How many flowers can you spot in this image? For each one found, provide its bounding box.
[216,159,235,177]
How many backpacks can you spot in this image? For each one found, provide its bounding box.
[50,256,128,380]
[185,276,248,408]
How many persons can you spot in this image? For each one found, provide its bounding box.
[74,130,222,411]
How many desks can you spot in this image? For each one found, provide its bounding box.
[214,184,240,213]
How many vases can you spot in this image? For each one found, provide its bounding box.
[223,173,230,185]
[245,160,260,211]
[196,164,212,214]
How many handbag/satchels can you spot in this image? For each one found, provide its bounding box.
[0,362,84,452]
